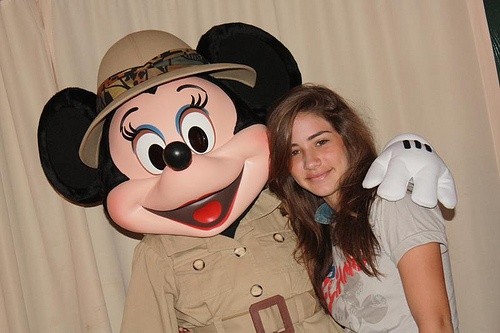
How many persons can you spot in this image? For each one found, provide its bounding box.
[37,21,457,333]
[266,83,459,333]
